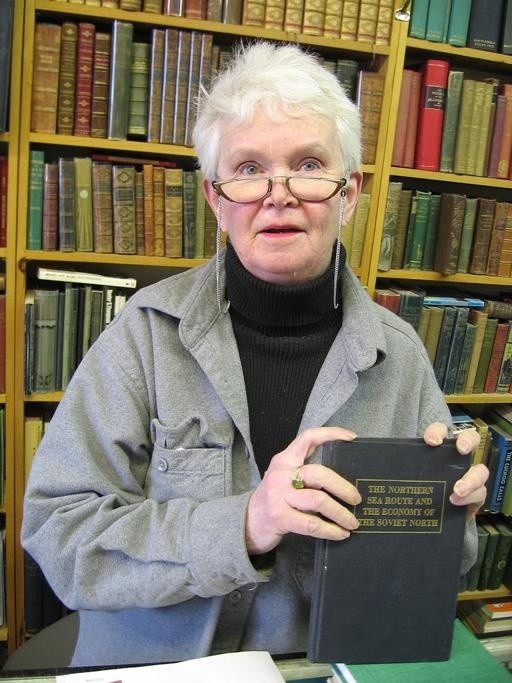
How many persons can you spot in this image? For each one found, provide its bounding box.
[22,42,492,672]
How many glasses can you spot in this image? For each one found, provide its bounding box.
[211,176,346,204]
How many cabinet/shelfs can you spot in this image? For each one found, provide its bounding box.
[1,0,512,659]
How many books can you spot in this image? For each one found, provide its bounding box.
[56,650,287,682]
[307,431,472,665]
[0,0,230,499]
[445,403,511,598]
[329,596,512,683]
[23,602,73,641]
[329,2,511,395]
[224,0,342,43]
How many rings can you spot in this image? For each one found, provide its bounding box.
[291,460,309,492]
